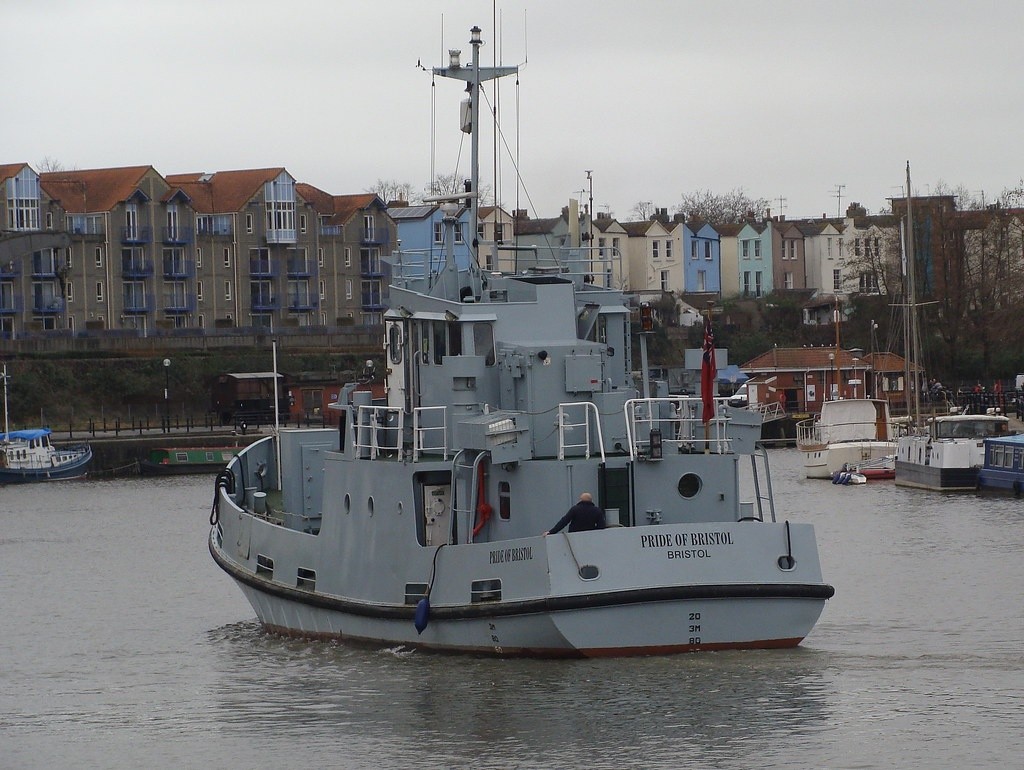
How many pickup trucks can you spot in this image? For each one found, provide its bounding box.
[667,394,695,418]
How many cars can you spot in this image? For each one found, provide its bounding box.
[950,386,997,404]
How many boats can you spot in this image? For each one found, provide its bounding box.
[972,432,1024,497]
[0,429,94,484]
[137,447,247,477]
[206,22,835,653]
[795,398,900,481]
[894,414,1009,490]
[833,454,896,486]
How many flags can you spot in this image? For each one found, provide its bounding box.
[701,317,716,423]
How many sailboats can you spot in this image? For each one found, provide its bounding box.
[867,160,958,435]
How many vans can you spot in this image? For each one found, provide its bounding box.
[729,381,748,407]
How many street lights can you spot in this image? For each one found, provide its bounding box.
[365,359,373,388]
[829,352,836,400]
[164,359,171,431]
[852,358,859,397]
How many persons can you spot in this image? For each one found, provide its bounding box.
[543,493,605,537]
[780,391,786,409]
